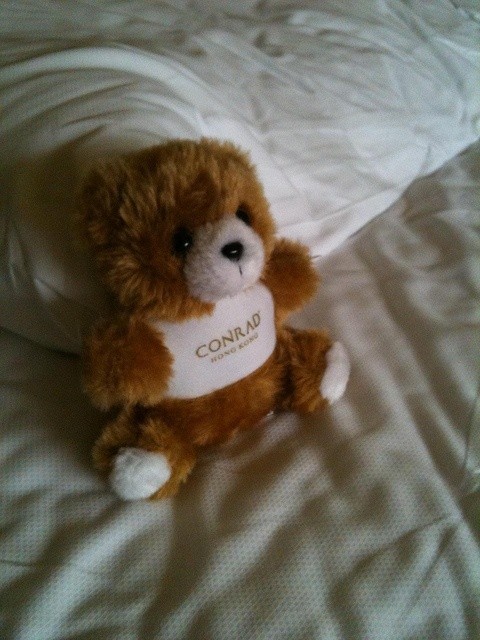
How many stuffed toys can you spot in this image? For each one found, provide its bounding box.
[82,144,350,500]
